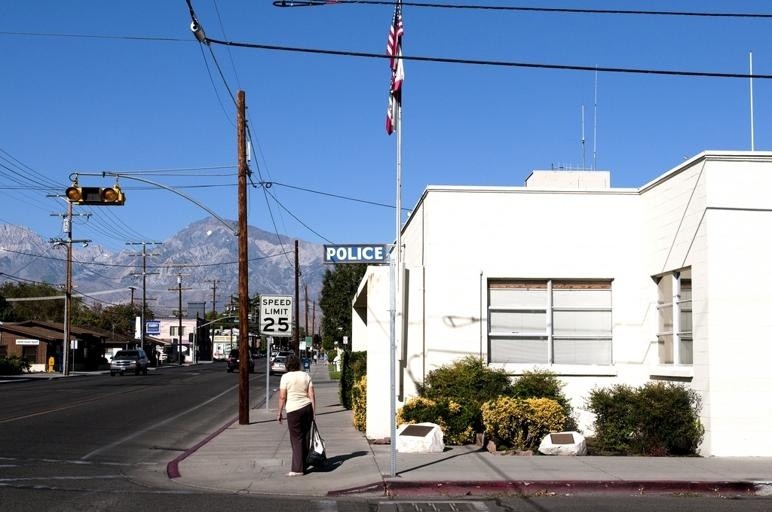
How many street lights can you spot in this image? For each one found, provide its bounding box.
[46,238,93,376]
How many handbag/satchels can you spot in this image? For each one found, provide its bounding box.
[305,419,329,470]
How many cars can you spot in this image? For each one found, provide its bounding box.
[270,349,317,375]
[253,347,267,359]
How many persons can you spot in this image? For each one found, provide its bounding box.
[313,352,317,364]
[277,353,317,478]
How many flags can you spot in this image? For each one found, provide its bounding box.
[384,5,404,137]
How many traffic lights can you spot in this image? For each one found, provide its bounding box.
[65,186,126,207]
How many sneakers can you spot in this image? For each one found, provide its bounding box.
[283,470,304,478]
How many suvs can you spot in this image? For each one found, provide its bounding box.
[111,349,149,377]
[227,348,256,373]
